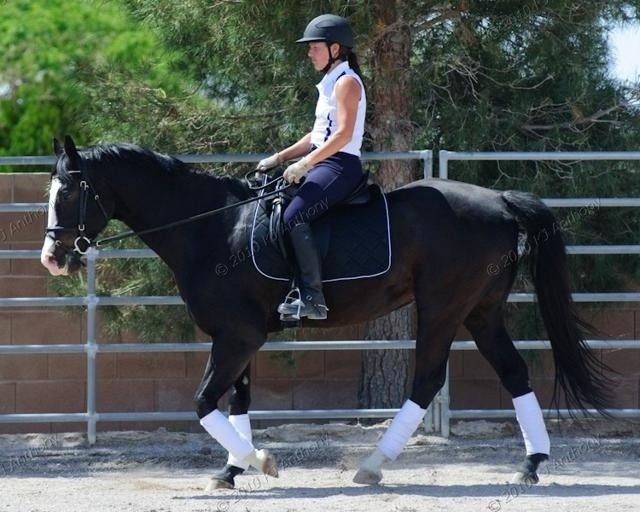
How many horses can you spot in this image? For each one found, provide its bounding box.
[38,133,629,490]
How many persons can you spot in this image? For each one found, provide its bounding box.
[251,10,369,322]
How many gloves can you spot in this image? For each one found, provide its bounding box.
[253,152,280,181]
[283,156,315,185]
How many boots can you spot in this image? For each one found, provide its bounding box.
[278,223,329,320]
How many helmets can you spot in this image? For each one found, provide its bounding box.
[295,14,354,51]
